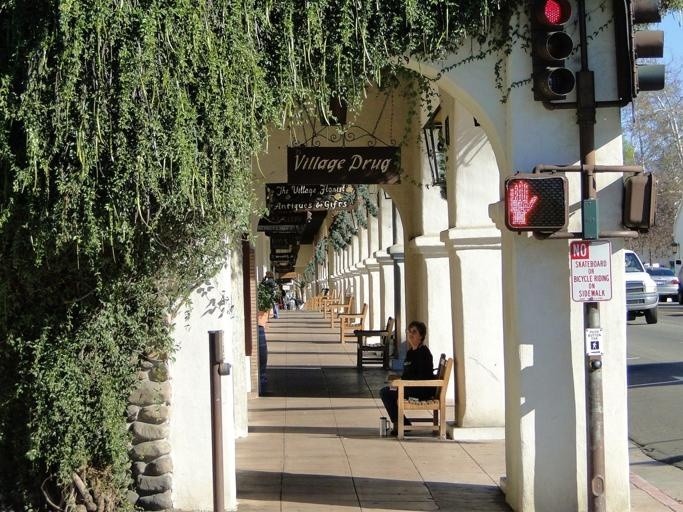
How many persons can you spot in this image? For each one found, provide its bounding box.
[378,320,436,437]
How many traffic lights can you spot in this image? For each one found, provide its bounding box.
[622,170,657,232]
[610,0,666,106]
[526,0,576,103]
[502,170,568,233]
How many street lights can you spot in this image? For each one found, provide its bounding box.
[669,240,678,277]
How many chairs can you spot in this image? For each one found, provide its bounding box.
[303,288,455,442]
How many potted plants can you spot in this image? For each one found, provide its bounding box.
[257,276,281,325]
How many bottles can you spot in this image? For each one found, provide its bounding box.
[380,417,390,438]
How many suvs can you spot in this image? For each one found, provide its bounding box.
[622,248,659,325]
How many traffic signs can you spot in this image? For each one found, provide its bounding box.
[566,239,611,303]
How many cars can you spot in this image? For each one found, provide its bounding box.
[641,264,678,302]
[673,265,682,305]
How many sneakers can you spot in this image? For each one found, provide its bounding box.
[390,417,412,437]
